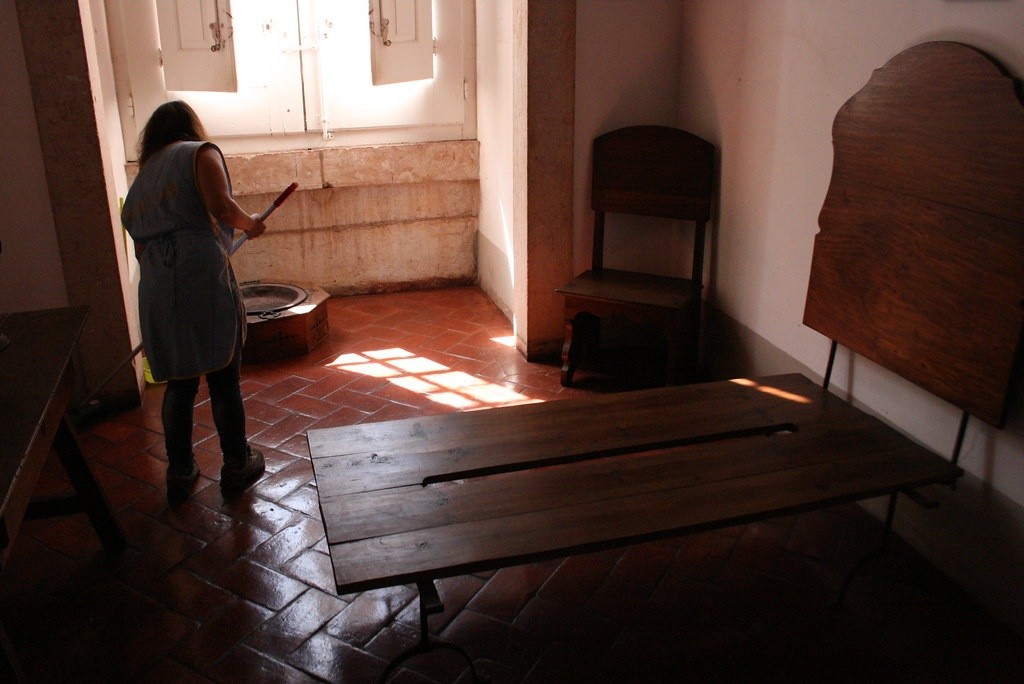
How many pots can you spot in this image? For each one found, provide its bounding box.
[238,279,308,319]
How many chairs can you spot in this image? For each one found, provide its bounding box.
[554,125,717,389]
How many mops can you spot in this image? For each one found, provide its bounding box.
[69,183,298,437]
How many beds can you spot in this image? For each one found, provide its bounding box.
[305,40,1024,684]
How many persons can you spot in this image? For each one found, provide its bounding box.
[122,102,264,503]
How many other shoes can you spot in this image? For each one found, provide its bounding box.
[165,459,199,499]
[221,444,265,493]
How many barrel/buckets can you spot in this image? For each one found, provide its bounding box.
[142,357,168,384]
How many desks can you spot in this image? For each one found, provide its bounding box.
[0,306,127,578]
